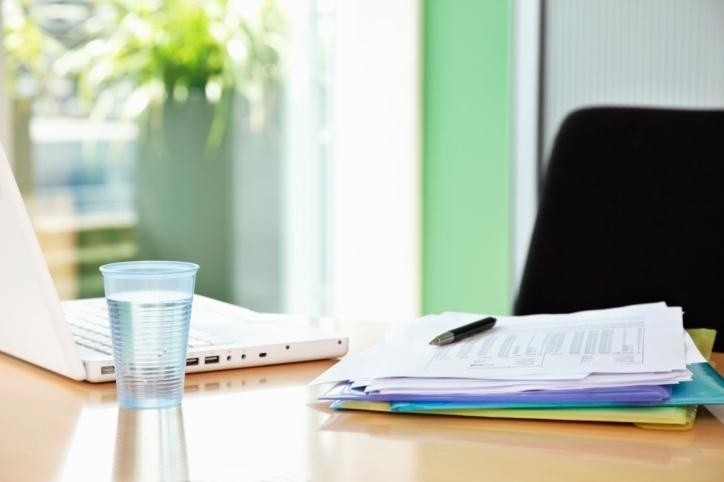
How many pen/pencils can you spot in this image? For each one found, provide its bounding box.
[428,316,497,346]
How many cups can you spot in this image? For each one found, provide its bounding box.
[99,260,200,410]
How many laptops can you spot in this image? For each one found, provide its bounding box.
[0,139,349,384]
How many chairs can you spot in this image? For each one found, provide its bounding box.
[515,106,718,352]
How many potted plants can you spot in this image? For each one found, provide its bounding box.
[79,2,281,302]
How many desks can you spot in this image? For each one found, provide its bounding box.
[0,344,720,482]
